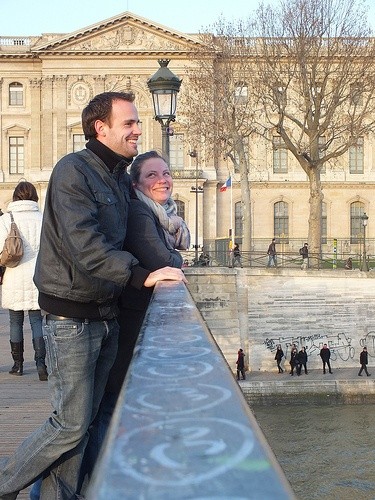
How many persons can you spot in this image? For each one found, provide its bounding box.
[301,243,309,270]
[29,150,190,500]
[320,344,333,374]
[236,348,246,380]
[274,345,284,374]
[184,259,189,267]
[267,239,277,268]
[357,346,371,377]
[345,258,352,270]
[229,243,243,268]
[0,92,190,500]
[288,344,308,377]
[199,246,210,266]
[0,182,50,382]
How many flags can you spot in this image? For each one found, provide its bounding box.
[220,177,231,192]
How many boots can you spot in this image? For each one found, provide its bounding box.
[32,337,49,381]
[9,338,25,376]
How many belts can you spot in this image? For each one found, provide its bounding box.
[41,314,74,321]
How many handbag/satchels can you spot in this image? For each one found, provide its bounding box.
[0,211,23,269]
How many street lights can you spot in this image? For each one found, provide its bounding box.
[146,59,183,171]
[361,212,369,271]
[189,150,204,267]
